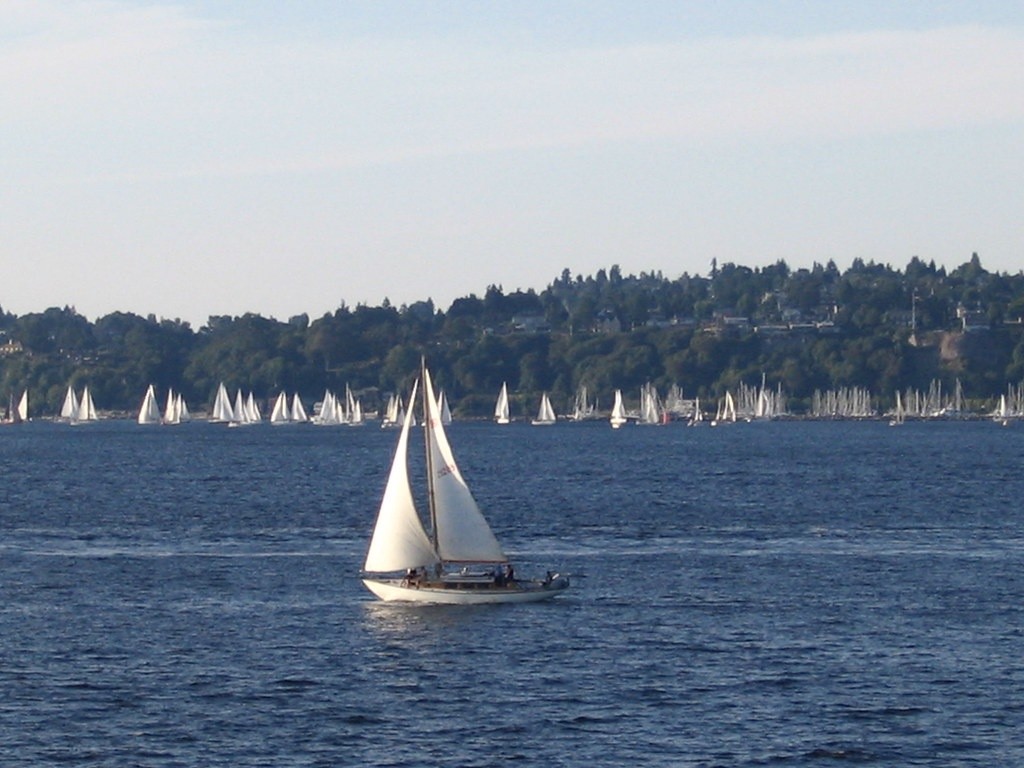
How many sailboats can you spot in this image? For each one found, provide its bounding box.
[0,375,1024,431]
[352,354,571,606]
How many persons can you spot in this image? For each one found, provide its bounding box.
[461,567,469,576]
[545,570,553,583]
[483,565,514,586]
[420,566,427,577]
